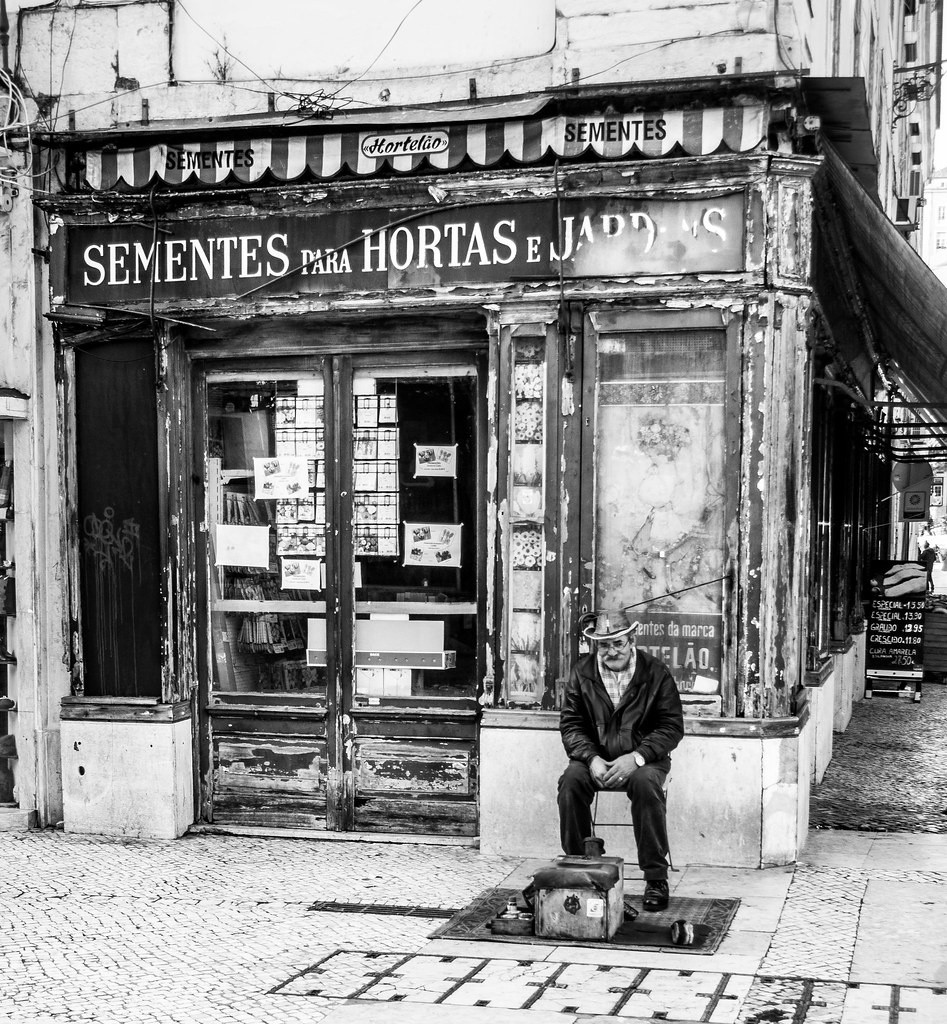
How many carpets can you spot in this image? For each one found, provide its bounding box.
[427,887,742,956]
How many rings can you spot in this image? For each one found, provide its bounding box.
[619,777,623,781]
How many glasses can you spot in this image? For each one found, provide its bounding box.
[597,637,631,651]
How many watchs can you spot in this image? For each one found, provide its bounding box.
[630,751,646,767]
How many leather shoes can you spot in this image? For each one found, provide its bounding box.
[643,880,669,911]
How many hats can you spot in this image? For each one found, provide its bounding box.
[584,609,639,641]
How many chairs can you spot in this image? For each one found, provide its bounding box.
[590,750,680,881]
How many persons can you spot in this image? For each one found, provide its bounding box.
[556,609,685,914]
[917,541,940,593]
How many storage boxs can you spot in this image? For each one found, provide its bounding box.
[528,851,625,944]
